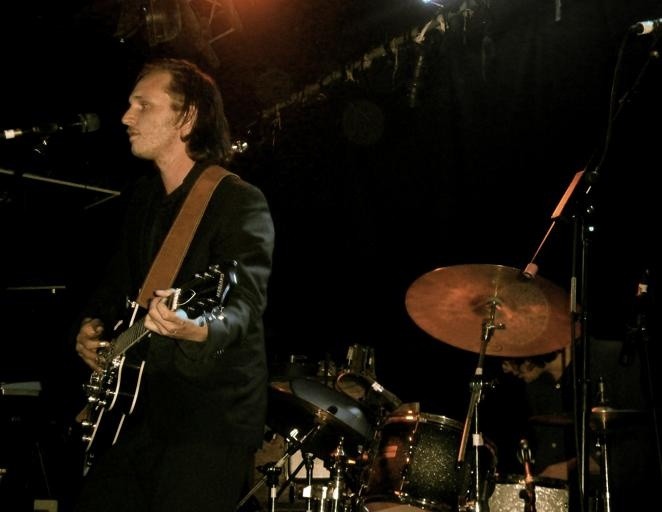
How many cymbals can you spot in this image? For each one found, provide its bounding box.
[290,375,376,438]
[337,368,403,410]
[406,263,582,355]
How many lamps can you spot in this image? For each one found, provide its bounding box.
[403,46,431,109]
[229,123,250,154]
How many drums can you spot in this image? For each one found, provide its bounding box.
[488,471,570,512]
[368,411,499,510]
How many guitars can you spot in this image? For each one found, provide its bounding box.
[71,263,239,463]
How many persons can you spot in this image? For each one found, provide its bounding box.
[47,55,277,512]
[500,333,641,487]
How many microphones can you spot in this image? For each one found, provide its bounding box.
[1,112,101,139]
